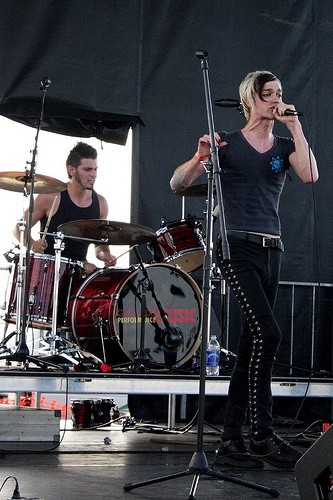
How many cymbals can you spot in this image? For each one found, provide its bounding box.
[173,182,217,198]
[57,220,157,246]
[0,171,68,195]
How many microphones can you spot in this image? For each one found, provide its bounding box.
[213,98,242,107]
[277,108,303,116]
[41,79,52,88]
[162,316,182,345]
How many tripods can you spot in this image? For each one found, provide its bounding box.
[0,87,112,372]
[112,51,280,500]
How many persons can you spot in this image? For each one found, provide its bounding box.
[170,71,318,469]
[14,142,117,279]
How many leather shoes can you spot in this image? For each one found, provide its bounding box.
[249,430,304,470]
[214,437,264,469]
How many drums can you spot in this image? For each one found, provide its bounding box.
[149,216,206,273]
[2,252,87,333]
[66,263,202,372]
[70,399,114,429]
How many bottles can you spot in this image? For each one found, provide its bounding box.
[206,335,220,376]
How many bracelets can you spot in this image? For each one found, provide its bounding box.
[195,152,209,160]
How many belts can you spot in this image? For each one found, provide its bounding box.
[218,229,285,251]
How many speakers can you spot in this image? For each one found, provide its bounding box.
[295,422,333,500]
